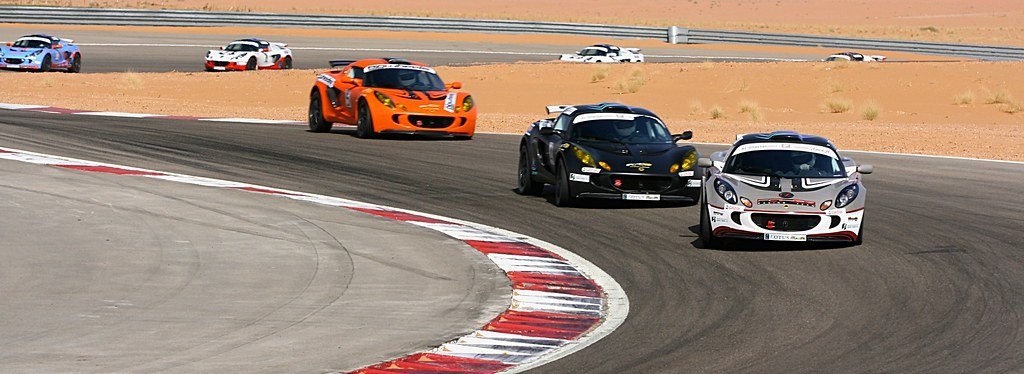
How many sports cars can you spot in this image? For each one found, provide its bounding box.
[1,32,81,73]
[559,43,644,64]
[205,37,295,71]
[516,102,702,205]
[696,130,877,247]
[308,57,480,138]
[824,50,887,64]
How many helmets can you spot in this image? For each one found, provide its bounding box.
[790,151,816,172]
[397,70,417,87]
[613,120,637,137]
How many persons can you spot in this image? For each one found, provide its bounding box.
[785,151,828,177]
[397,69,427,89]
[612,118,644,142]
